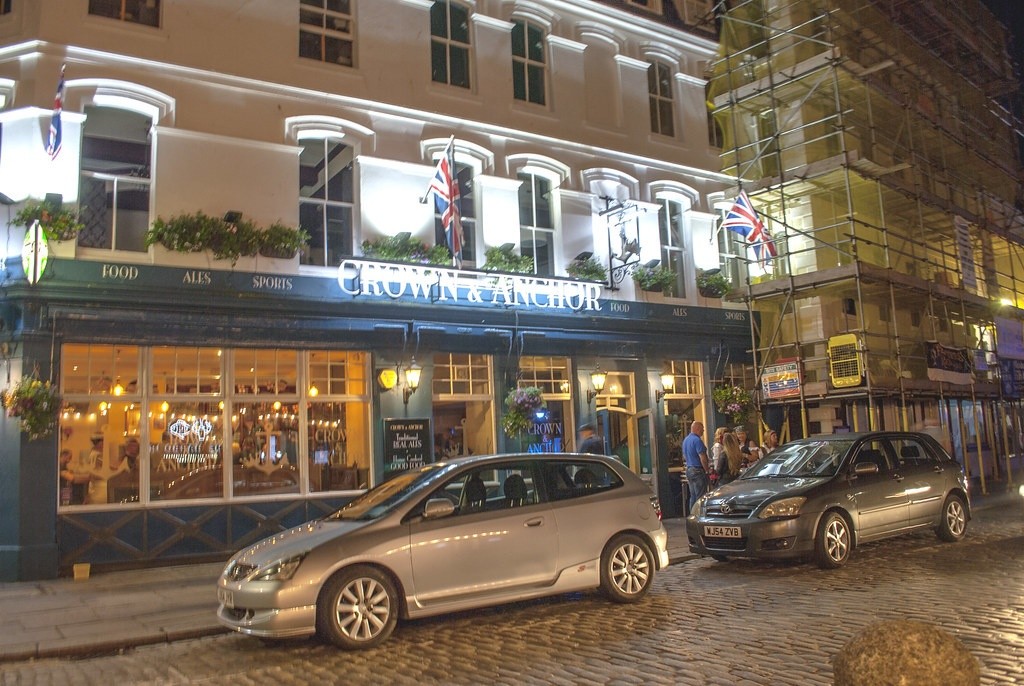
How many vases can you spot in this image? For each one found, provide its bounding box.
[257,239,298,258]
[210,235,249,255]
[160,233,203,251]
[700,288,723,298]
[640,280,662,292]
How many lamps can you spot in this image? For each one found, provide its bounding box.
[587,362,607,402]
[707,269,721,274]
[394,232,411,241]
[46,193,62,206]
[656,369,675,402]
[645,260,660,268]
[575,252,593,260]
[224,211,242,222]
[403,356,422,404]
[501,243,515,252]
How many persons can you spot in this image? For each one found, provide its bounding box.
[150,431,179,468]
[716,433,742,487]
[733,425,759,467]
[121,440,139,471]
[279,431,298,465]
[682,421,710,511]
[60,449,75,487]
[233,437,262,464]
[712,425,729,471]
[758,429,778,460]
[578,423,604,454]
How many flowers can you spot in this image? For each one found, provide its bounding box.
[697,268,731,295]
[498,387,547,437]
[482,246,534,272]
[365,236,449,266]
[713,384,753,426]
[8,200,87,241]
[631,264,679,291]
[0,375,64,439]
[565,258,607,281]
[143,209,312,277]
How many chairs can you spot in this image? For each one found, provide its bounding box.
[899,446,921,457]
[574,469,596,487]
[504,474,527,507]
[466,478,486,504]
[861,449,881,464]
[822,454,833,465]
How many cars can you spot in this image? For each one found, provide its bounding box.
[684,430,973,569]
[118,460,315,505]
[213,448,672,652]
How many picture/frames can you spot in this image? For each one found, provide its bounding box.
[124,407,142,436]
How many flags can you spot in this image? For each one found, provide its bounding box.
[432,143,465,269]
[46,73,64,161]
[722,190,778,269]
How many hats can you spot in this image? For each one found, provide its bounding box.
[577,423,594,431]
